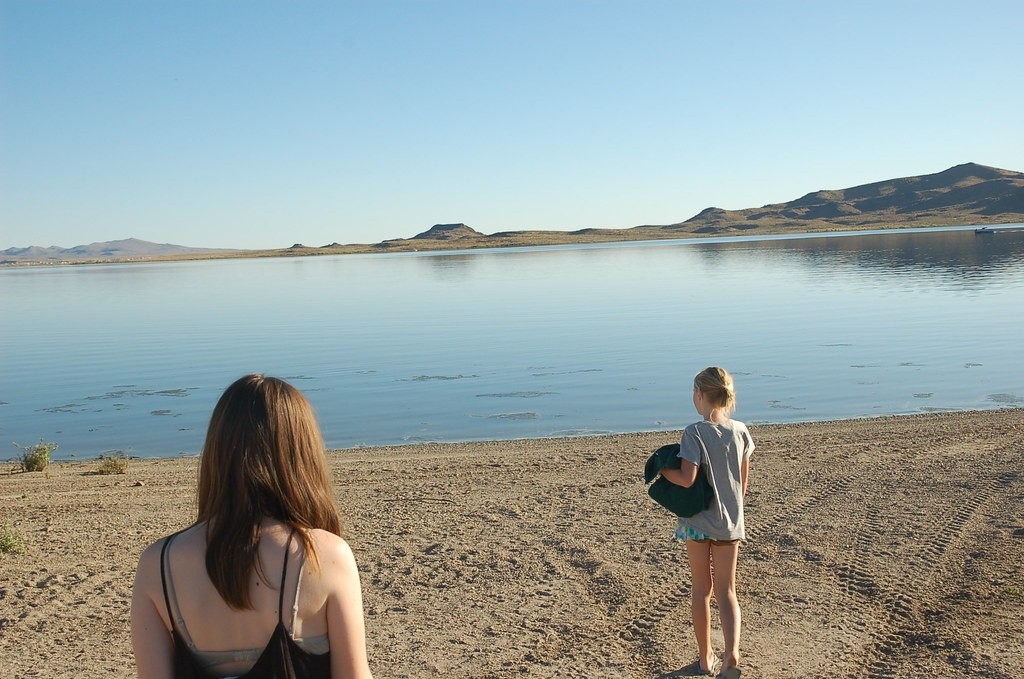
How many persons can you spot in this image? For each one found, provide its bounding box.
[657,366,755,679]
[130,376,375,679]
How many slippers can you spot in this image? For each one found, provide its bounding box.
[698,654,719,673]
[716,666,741,679]
